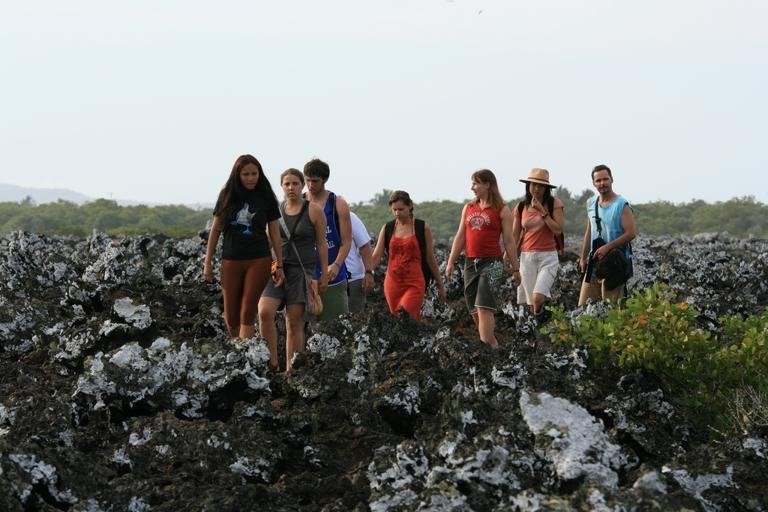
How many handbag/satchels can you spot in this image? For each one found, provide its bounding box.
[590,237,631,291]
[305,278,323,315]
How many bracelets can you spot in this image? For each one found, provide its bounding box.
[277,267,282,268]
[608,243,613,250]
[437,283,444,289]
[542,213,548,219]
[366,269,374,275]
[334,262,341,269]
[514,269,519,272]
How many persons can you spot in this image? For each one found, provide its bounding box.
[204,156,286,341]
[372,191,446,321]
[578,164,635,306]
[445,169,521,349]
[302,159,352,322]
[503,168,565,323]
[344,211,374,313]
[257,168,328,374]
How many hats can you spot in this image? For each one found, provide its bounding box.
[519,168,556,188]
